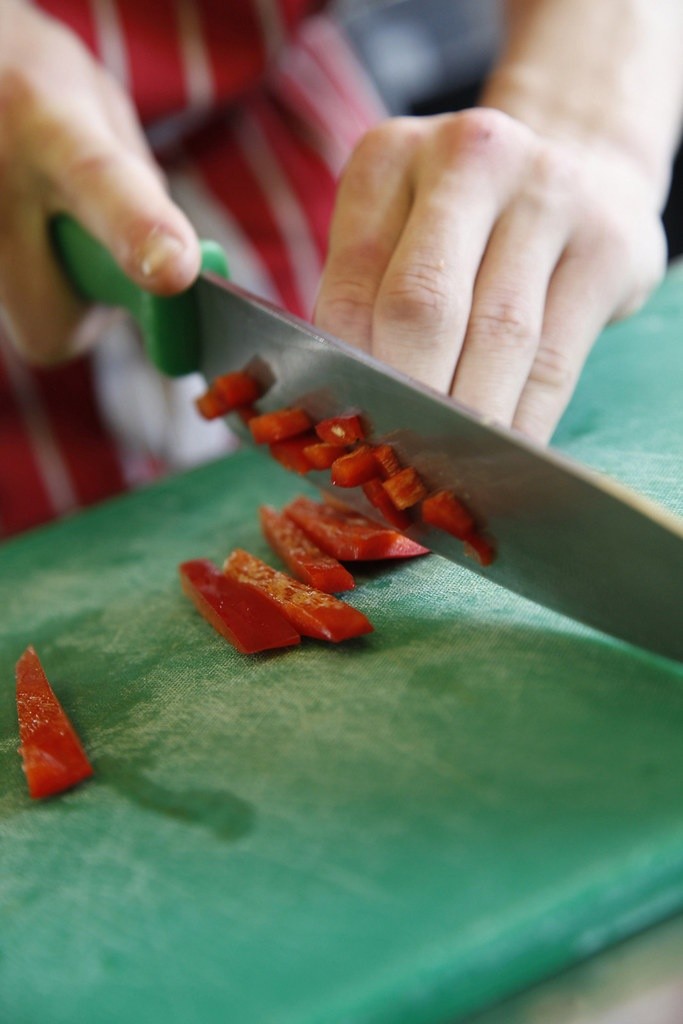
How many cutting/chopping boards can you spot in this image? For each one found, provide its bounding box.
[1,262,683,1024]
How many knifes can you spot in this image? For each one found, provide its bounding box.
[50,210,683,666]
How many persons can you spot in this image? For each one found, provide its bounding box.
[0,0,683,542]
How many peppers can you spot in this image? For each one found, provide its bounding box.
[180,373,493,655]
[15,638,92,798]
